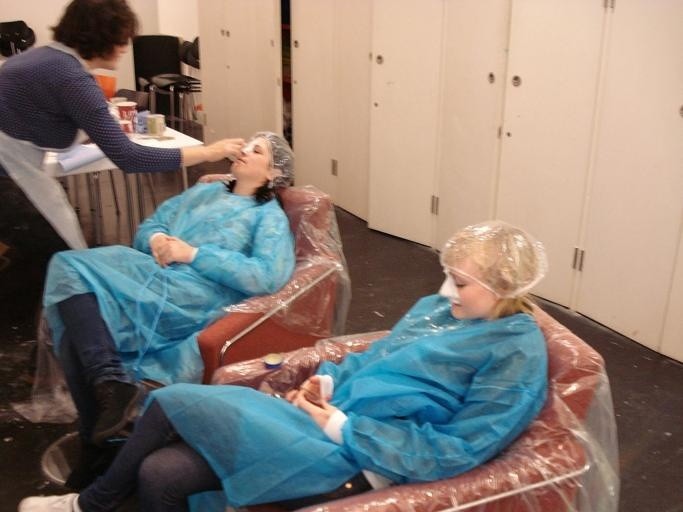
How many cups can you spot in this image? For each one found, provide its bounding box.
[109,97,165,135]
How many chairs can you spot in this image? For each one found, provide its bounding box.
[41,175,341,487]
[215,298,604,512]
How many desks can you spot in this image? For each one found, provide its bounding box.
[42,126,204,249]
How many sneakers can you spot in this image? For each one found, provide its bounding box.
[90,378,140,438]
[17,493,78,511]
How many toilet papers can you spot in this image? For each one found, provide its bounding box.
[56,138,110,171]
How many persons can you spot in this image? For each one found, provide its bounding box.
[43,130,318,485]
[1,0,253,325]
[12,224,545,511]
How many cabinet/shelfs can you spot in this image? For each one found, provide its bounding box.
[334,1,442,247]
[196,0,248,191]
[248,0,334,223]
[440,1,609,309]
[575,2,683,365]
